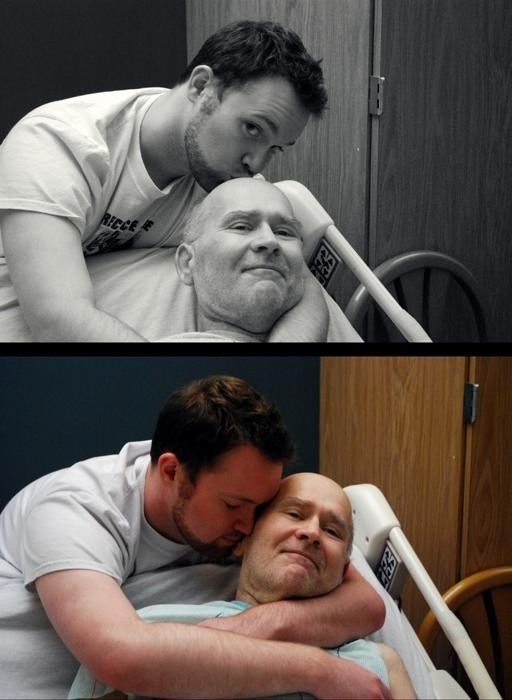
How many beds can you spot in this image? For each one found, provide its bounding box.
[0,180,435,344]
[0,484,503,700]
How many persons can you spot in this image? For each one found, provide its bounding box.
[1,374,395,699]
[156,177,309,342]
[69,470,418,700]
[0,21,328,346]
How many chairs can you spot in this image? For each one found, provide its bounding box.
[417,566,512,700]
[345,250,489,344]
